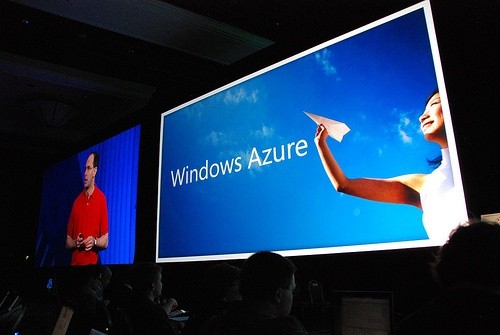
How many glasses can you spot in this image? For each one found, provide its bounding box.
[95,277,103,282]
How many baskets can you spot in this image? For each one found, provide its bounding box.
[333,296,390,335]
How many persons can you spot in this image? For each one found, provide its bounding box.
[313,89,459,239]
[395,221,500,335]
[182,263,242,335]
[0,263,182,335]
[199,250,310,335]
[65,151,108,265]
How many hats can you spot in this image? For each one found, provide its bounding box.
[102,266,113,277]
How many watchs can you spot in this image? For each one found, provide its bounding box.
[94,237,98,246]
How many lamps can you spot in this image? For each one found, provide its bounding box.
[25,98,82,129]
